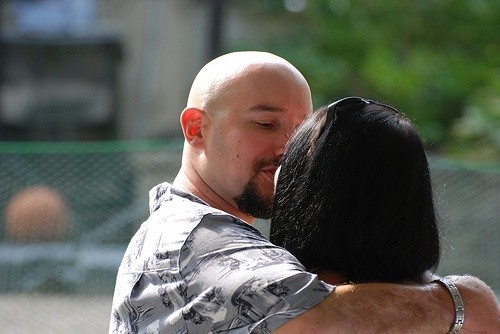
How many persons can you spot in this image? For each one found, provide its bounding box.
[269,97,443,285]
[106,51,500,334]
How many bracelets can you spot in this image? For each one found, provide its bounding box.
[429,277,465,334]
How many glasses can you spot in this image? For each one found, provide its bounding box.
[320,97,405,134]
